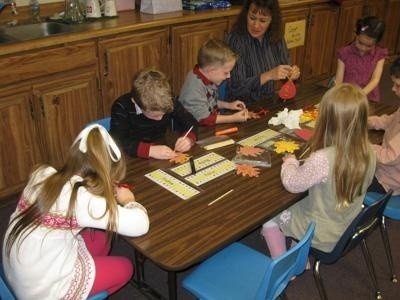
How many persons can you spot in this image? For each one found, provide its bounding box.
[334,16,388,105]
[353,57,400,195]
[106,68,196,162]
[2,122,154,300]
[227,1,302,109]
[178,39,250,124]
[264,82,379,279]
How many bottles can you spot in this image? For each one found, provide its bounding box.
[63,0,85,26]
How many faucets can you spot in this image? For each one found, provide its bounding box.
[30,0,42,24]
[0,2,20,17]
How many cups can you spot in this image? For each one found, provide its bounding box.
[104,0,118,16]
[86,0,103,19]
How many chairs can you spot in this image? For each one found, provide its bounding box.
[364,188,400,283]
[0,263,108,300]
[181,221,317,300]
[278,187,395,299]
[85,114,112,136]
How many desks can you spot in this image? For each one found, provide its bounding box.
[105,81,399,300]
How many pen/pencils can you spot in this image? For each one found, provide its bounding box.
[173,126,194,154]
[207,188,235,207]
[298,146,312,160]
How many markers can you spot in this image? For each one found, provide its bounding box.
[215,126,239,136]
[189,157,196,175]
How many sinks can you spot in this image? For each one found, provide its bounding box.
[15,19,81,41]
[0,25,21,46]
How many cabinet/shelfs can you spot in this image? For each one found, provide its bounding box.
[0,32,100,206]
[337,0,400,56]
[168,19,227,101]
[276,5,338,88]
[97,26,173,128]
